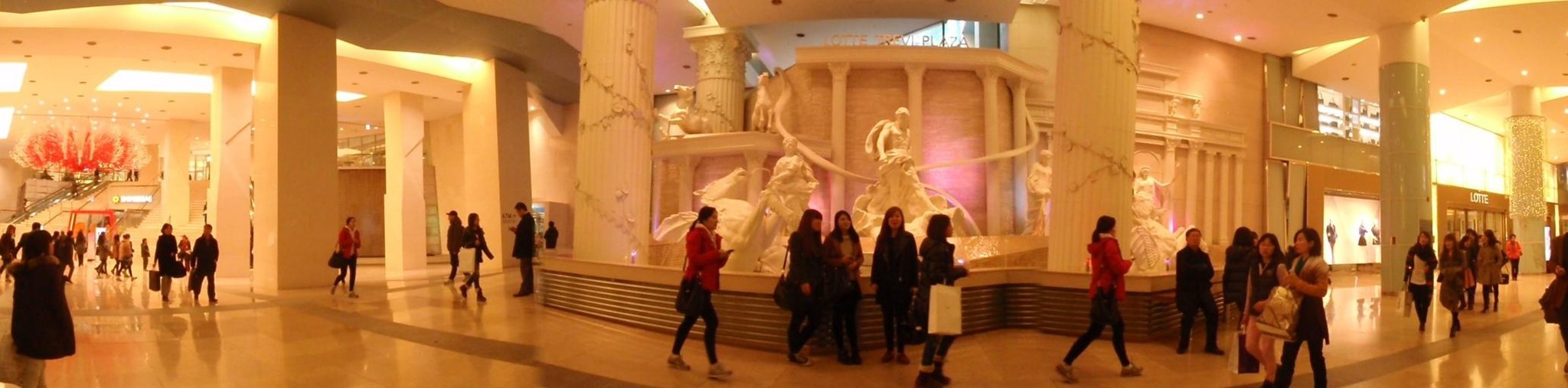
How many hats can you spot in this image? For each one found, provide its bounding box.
[446,210,457,216]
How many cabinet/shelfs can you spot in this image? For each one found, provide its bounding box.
[1317,102,1381,145]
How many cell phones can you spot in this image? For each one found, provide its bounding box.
[727,249,734,254]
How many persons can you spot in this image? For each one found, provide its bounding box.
[542,221,559,248]
[781,206,826,365]
[2,165,221,386]
[443,211,467,287]
[1215,220,1568,387]
[822,209,867,367]
[508,201,534,298]
[1171,228,1225,355]
[329,216,362,299]
[1129,163,1182,236]
[664,206,734,379]
[911,211,970,387]
[1051,215,1145,384]
[764,137,820,227]
[865,107,943,217]
[871,207,918,367]
[1024,147,1056,238]
[453,213,495,302]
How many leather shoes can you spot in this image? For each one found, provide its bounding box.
[1450,319,1460,337]
[514,290,529,297]
[1459,299,1498,312]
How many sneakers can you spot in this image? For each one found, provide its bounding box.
[331,285,336,295]
[476,295,486,302]
[666,355,689,370]
[1204,346,1224,356]
[708,363,731,377]
[788,354,810,365]
[444,278,454,284]
[349,291,359,298]
[880,352,895,363]
[459,285,468,298]
[898,352,909,363]
[837,350,861,365]
[1056,362,1079,383]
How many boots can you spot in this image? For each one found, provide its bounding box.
[916,357,950,388]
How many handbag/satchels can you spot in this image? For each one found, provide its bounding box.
[328,250,346,268]
[1228,332,1261,374]
[773,276,801,310]
[1120,363,1143,377]
[1494,251,1509,264]
[928,284,962,336]
[1255,286,1303,342]
[675,276,704,317]
[1500,274,1509,284]
[458,248,475,273]
[150,269,161,293]
[1396,290,1412,317]
[1092,285,1120,325]
[171,261,185,278]
